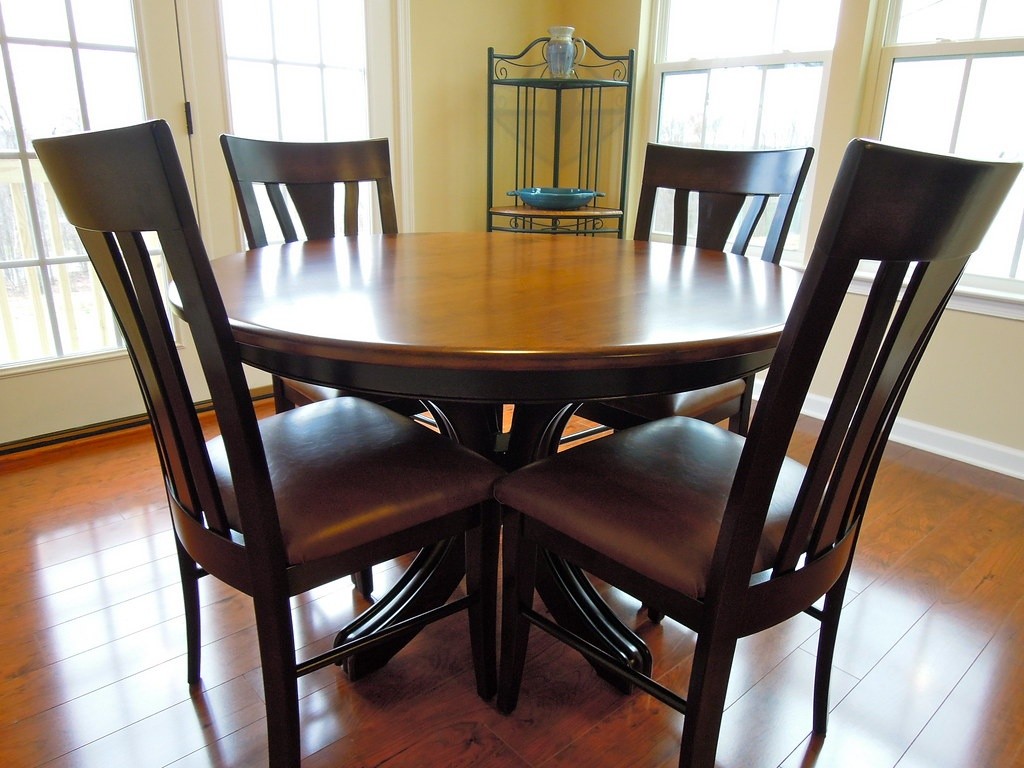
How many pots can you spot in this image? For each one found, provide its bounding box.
[506,187,606,209]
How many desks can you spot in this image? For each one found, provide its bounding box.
[165,225,804,699]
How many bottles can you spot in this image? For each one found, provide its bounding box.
[547,27,586,77]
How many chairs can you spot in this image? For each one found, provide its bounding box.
[496,137,1024,768]
[558,143,814,454]
[30,119,507,768]
[217,129,439,598]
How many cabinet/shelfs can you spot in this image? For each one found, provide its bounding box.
[485,35,635,239]
